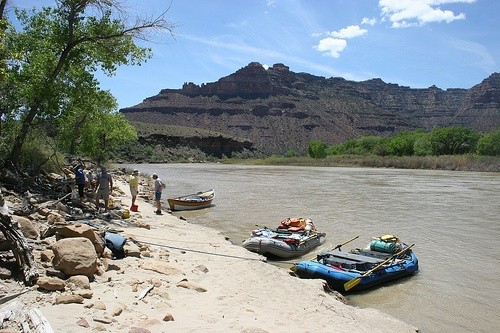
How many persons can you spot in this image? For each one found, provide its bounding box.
[129,169,139,210]
[152,172,166,214]
[74,161,86,203]
[93,167,113,212]
[86,167,94,190]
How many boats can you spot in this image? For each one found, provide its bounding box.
[167,188,216,211]
[293,234,419,293]
[240,218,325,258]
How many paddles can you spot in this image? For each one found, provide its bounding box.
[254,224,272,229]
[299,233,326,246]
[289,235,360,271]
[343,243,416,291]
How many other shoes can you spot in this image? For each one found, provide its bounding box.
[154,209,161,215]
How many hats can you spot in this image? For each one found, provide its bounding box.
[131,169,140,175]
